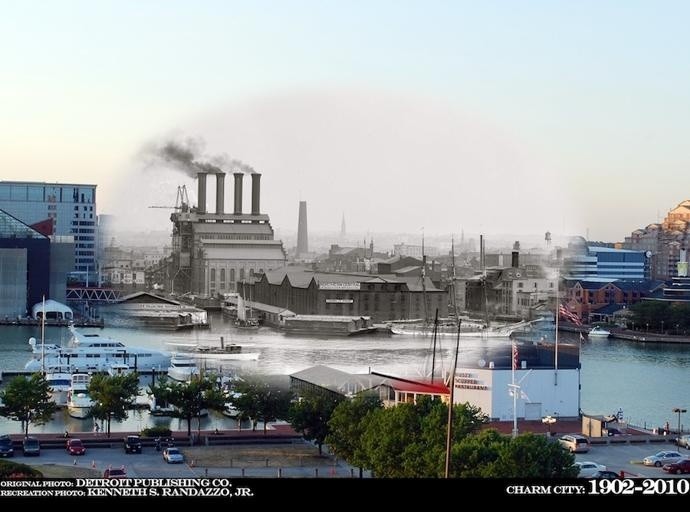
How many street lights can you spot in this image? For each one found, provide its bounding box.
[542,415,557,437]
[671,405,688,453]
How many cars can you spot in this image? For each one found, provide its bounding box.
[674,434,690,448]
[583,469,626,478]
[662,456,690,474]
[65,438,87,458]
[562,460,608,479]
[121,435,144,454]
[21,435,42,455]
[101,466,129,479]
[162,446,184,465]
[642,448,688,468]
[0,436,18,457]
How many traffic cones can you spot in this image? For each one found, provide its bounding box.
[92,459,97,468]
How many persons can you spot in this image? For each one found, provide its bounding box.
[95,423,100,435]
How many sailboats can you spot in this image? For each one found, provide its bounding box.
[232,281,260,330]
[382,235,525,340]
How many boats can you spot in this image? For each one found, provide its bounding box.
[42,349,260,422]
[582,326,614,340]
[166,335,261,362]
[23,324,172,373]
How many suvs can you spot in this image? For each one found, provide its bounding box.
[554,433,592,454]
[154,435,178,451]
